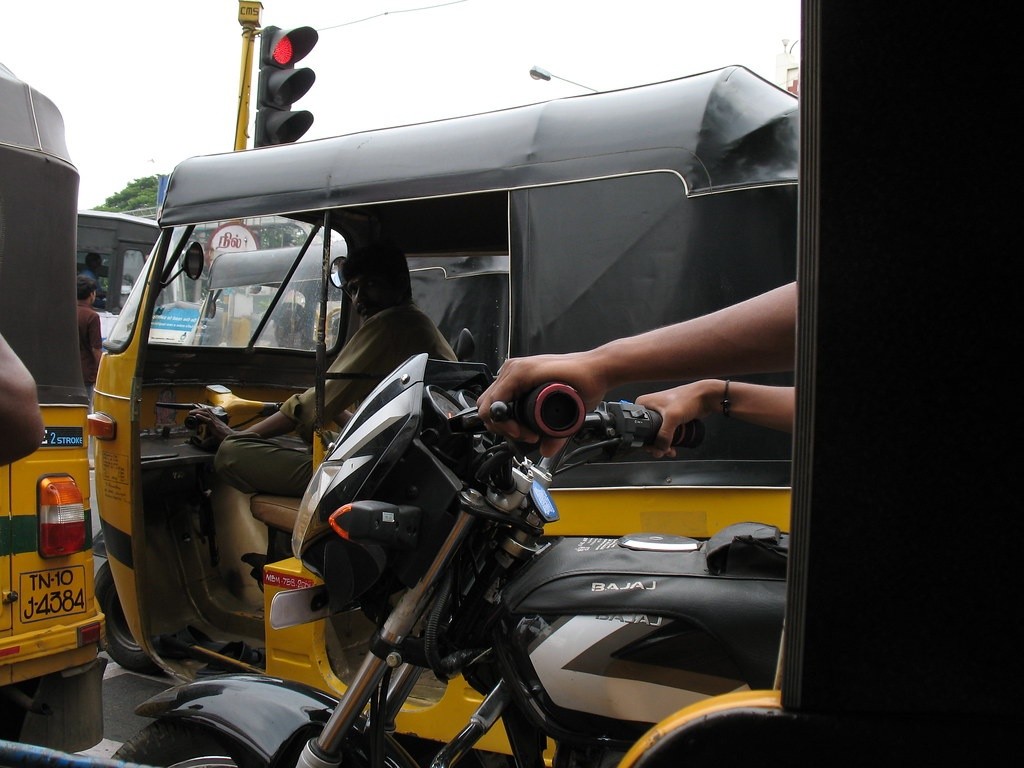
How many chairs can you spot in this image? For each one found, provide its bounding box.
[250,492,303,531]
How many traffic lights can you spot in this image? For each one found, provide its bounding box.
[254,25,318,146]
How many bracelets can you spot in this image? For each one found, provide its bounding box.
[720,380,729,418]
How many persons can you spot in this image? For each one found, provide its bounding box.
[476,282,801,456]
[0,332,47,466]
[79,252,106,309]
[76,274,103,399]
[189,233,459,566]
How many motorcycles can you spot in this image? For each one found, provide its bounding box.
[0,59,511,745]
[111,0,1024,768]
[92,61,802,676]
